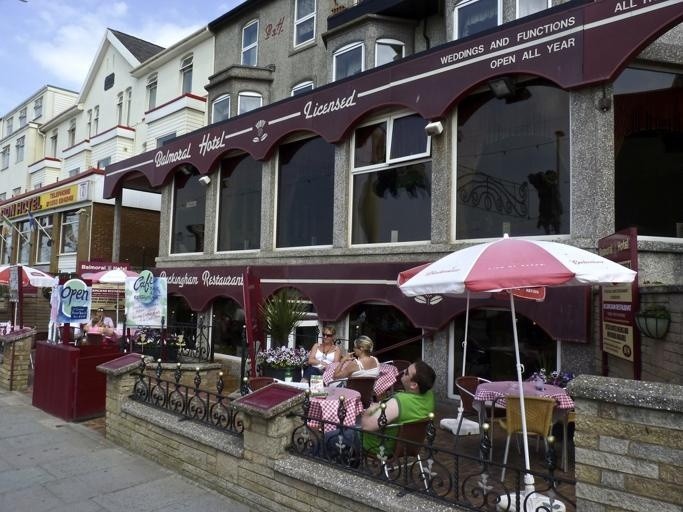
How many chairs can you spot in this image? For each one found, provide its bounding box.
[303,359,433,493]
[455,375,576,483]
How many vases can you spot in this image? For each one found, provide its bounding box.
[263,367,302,383]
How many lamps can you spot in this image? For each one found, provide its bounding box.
[181,165,193,174]
[490,76,517,100]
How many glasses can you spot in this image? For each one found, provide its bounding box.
[322,332,334,337]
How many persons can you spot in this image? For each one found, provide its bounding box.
[82,317,112,341]
[87,306,113,328]
[333,334,380,387]
[321,358,436,470]
[303,324,341,381]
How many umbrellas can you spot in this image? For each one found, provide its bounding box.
[394,233,638,486]
[90,267,140,326]
[0,263,55,329]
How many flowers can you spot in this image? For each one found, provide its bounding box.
[256,345,309,368]
